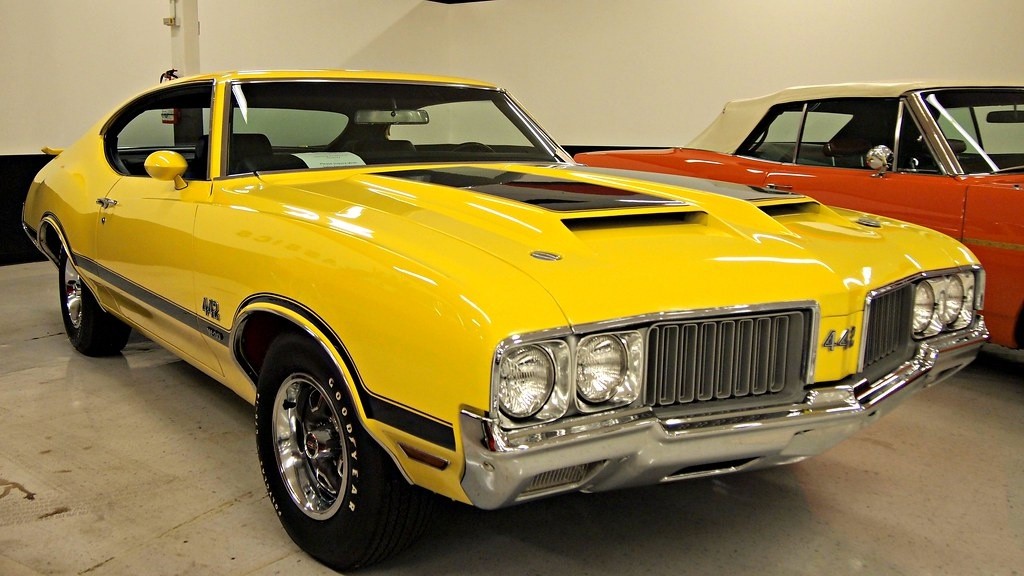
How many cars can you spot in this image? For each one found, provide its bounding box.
[572,80,1024,356]
[22,68,988,571]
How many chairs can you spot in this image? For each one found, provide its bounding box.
[920,139,969,176]
[820,135,872,168]
[194,132,273,180]
[351,138,417,165]
